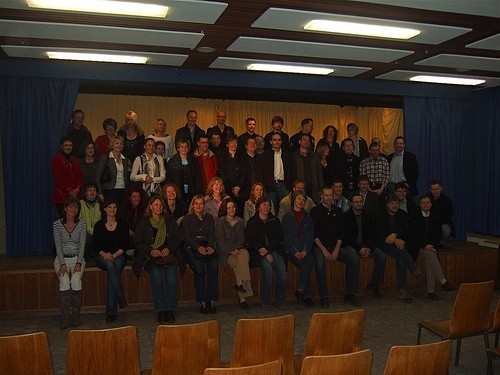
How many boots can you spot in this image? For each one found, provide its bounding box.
[58,289,82,330]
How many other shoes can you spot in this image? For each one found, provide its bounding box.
[411,270,425,282]
[263,305,289,310]
[240,302,248,309]
[322,297,330,308]
[343,294,363,307]
[429,293,439,301]
[442,283,456,290]
[107,315,116,323]
[367,284,383,298]
[232,285,246,294]
[118,297,128,308]
[157,311,175,326]
[401,289,411,303]
[294,291,315,307]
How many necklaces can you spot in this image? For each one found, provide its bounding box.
[109,225,113,226]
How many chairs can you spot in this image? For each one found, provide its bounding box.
[0,279,500,375]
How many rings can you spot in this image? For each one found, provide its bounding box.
[149,181,151,182]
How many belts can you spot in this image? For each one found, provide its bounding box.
[371,182,380,187]
[274,180,284,183]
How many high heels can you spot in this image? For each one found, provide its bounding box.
[199,301,216,314]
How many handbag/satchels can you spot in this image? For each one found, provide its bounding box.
[192,241,219,258]
[129,156,143,197]
[100,153,111,185]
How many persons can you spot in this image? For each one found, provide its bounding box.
[53,109,455,329]
